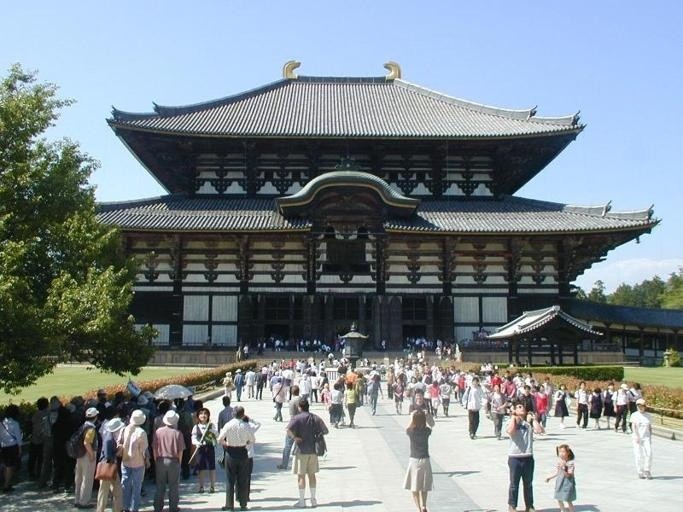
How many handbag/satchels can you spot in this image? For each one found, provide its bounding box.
[463,399,468,411]
[273,397,275,402]
[226,382,231,388]
[94,462,119,481]
[315,433,326,456]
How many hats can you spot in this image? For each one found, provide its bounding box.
[197,408,211,419]
[142,391,153,398]
[97,389,108,395]
[235,369,242,373]
[636,398,646,406]
[225,372,232,376]
[137,395,148,406]
[104,417,125,433]
[130,409,146,425]
[162,410,180,427]
[85,407,100,418]
[621,383,629,391]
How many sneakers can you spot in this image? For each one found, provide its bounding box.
[210,487,215,493]
[240,507,249,510]
[199,486,205,492]
[292,501,307,508]
[311,500,318,508]
[222,506,234,511]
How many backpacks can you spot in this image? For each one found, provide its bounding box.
[65,426,86,459]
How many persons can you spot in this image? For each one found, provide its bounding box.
[301,340,383,428]
[575,382,642,434]
[218,396,261,512]
[404,409,435,512]
[221,337,311,422]
[286,396,329,507]
[545,443,576,512]
[451,360,572,439]
[277,386,301,468]
[629,399,652,479]
[506,401,544,512]
[381,337,460,416]
[1,389,218,512]
